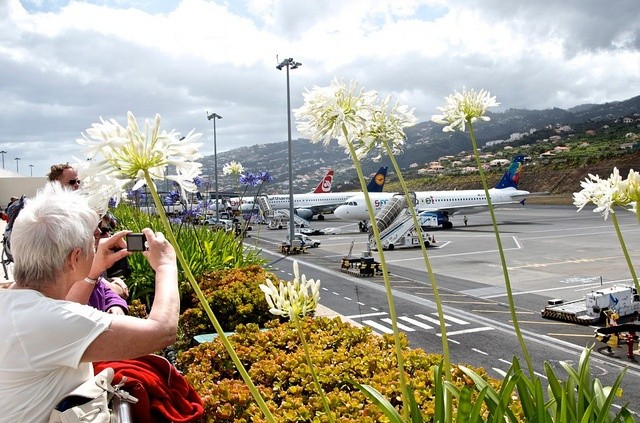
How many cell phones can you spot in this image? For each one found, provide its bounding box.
[126,233,148,252]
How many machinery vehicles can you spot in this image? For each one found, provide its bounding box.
[268,210,309,229]
[370,214,438,250]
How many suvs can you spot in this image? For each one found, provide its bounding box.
[287,233,321,247]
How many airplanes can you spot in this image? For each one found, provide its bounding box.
[210,168,334,214]
[334,155,550,232]
[238,166,389,220]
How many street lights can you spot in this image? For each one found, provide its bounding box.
[207,112,222,222]
[276,58,302,244]
[29,165,34,176]
[14,157,21,172]
[0,151,7,169]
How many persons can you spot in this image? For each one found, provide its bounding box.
[93,212,115,251]
[4,195,19,213]
[46,160,80,191]
[88,280,129,316]
[0,181,181,423]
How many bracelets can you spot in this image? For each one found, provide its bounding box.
[85,276,101,284]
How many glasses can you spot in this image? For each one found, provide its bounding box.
[66,180,81,185]
[100,227,111,235]
